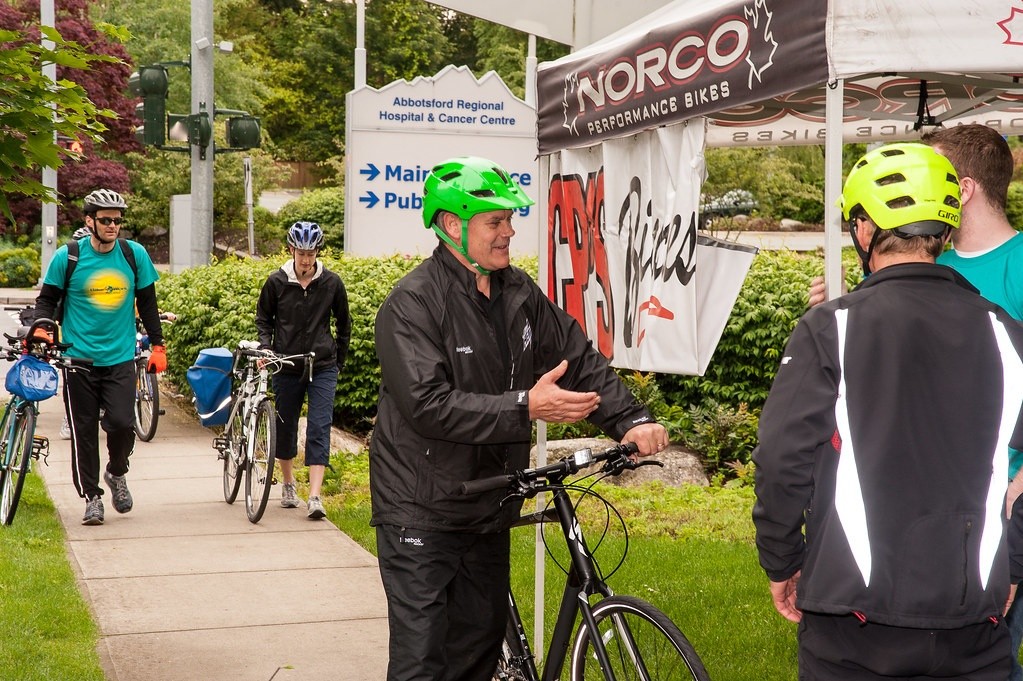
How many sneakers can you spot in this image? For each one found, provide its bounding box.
[60,416,71,440]
[280,480,300,508]
[81,494,105,525]
[306,496,326,518]
[103,471,134,514]
[99,408,106,420]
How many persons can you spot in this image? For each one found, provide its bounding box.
[255,220,351,520]
[370,157,669,680]
[29,189,166,525]
[61,226,91,440]
[749,142,1023,681]
[809,125,1023,661]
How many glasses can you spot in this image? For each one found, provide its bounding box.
[95,217,123,225]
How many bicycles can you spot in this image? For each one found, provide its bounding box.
[212,340,315,523]
[461,442,713,681]
[0,306,166,526]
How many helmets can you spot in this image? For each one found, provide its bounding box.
[422,157,536,228]
[72,226,91,240]
[287,221,324,251]
[834,142,962,241]
[83,188,128,212]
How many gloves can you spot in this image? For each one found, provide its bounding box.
[145,345,168,374]
[22,327,54,348]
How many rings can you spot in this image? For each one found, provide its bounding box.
[657,442,664,447]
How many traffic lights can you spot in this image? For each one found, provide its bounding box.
[129,65,169,146]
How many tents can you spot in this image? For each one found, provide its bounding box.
[531,0,1023,668]
